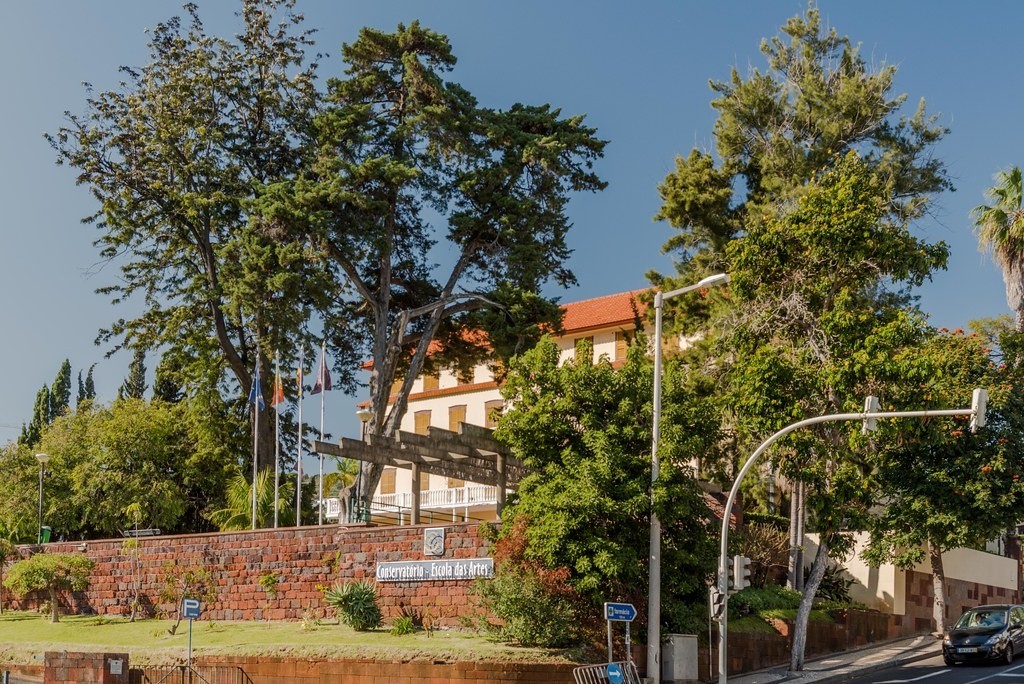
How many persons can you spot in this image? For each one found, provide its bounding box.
[998,612,1013,626]
[970,614,988,627]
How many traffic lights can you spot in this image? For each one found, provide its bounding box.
[732,554,752,590]
[863,396,883,433]
[968,388,990,428]
[710,586,726,621]
[717,556,733,590]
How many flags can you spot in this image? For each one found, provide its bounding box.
[248,346,331,412]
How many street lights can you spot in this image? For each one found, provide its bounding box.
[643,271,730,684]
[355,409,375,523]
[35,453,51,545]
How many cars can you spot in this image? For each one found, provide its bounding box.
[941,602,1024,665]
